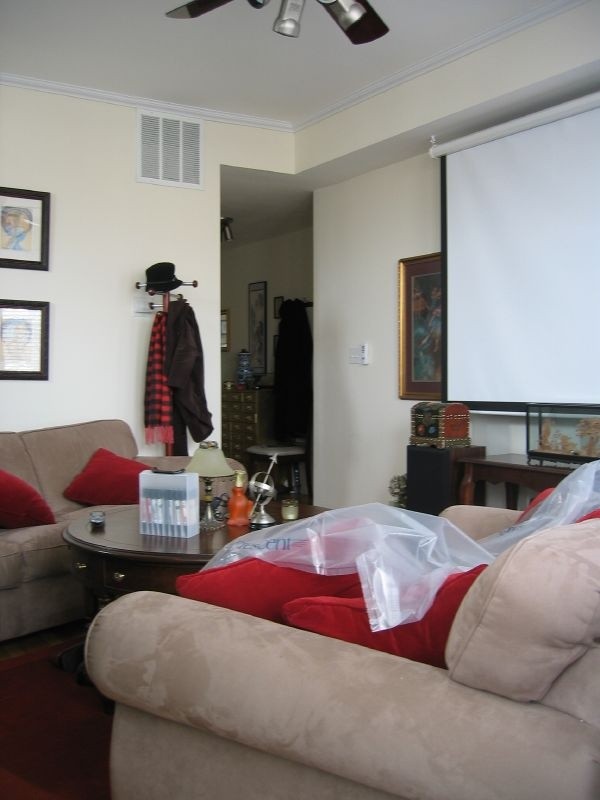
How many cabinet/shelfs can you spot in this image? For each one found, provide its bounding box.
[221,385,275,482]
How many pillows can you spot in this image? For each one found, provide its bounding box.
[176,515,369,626]
[0,468,56,530]
[61,448,159,506]
[281,560,490,671]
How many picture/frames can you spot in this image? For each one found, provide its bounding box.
[247,280,268,376]
[0,299,49,382]
[396,250,443,402]
[0,187,51,271]
[273,295,285,319]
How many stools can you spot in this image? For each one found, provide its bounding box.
[244,442,311,505]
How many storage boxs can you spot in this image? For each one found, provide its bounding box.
[525,400,600,467]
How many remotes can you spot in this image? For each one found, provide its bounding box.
[90,511,104,527]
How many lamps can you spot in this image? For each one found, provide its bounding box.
[247,0,367,39]
[182,439,236,529]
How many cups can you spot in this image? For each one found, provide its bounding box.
[224,382,234,387]
[281,498,299,521]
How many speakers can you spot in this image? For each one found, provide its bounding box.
[408,445,485,515]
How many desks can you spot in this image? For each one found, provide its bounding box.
[456,450,585,511]
[404,443,488,517]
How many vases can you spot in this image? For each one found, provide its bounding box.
[236,350,254,385]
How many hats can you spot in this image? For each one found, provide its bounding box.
[146,262,183,293]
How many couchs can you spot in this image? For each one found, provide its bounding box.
[84,484,600,800]
[0,417,248,641]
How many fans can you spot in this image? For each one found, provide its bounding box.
[165,0,391,46]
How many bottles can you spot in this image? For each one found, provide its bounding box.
[226,470,250,526]
[238,352,253,384]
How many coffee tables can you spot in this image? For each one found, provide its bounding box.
[60,500,334,635]
